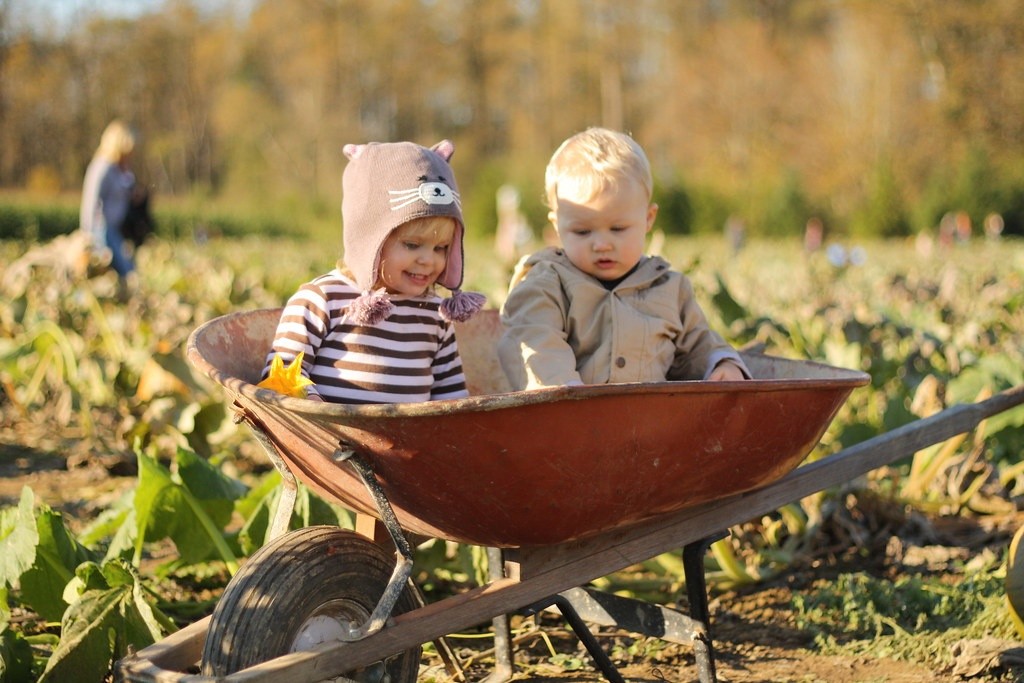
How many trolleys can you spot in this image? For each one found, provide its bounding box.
[112,302,1024,683]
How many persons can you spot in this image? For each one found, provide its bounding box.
[80,122,146,302]
[261,140,487,405]
[497,129,753,392]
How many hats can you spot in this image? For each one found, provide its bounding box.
[340,139,486,324]
